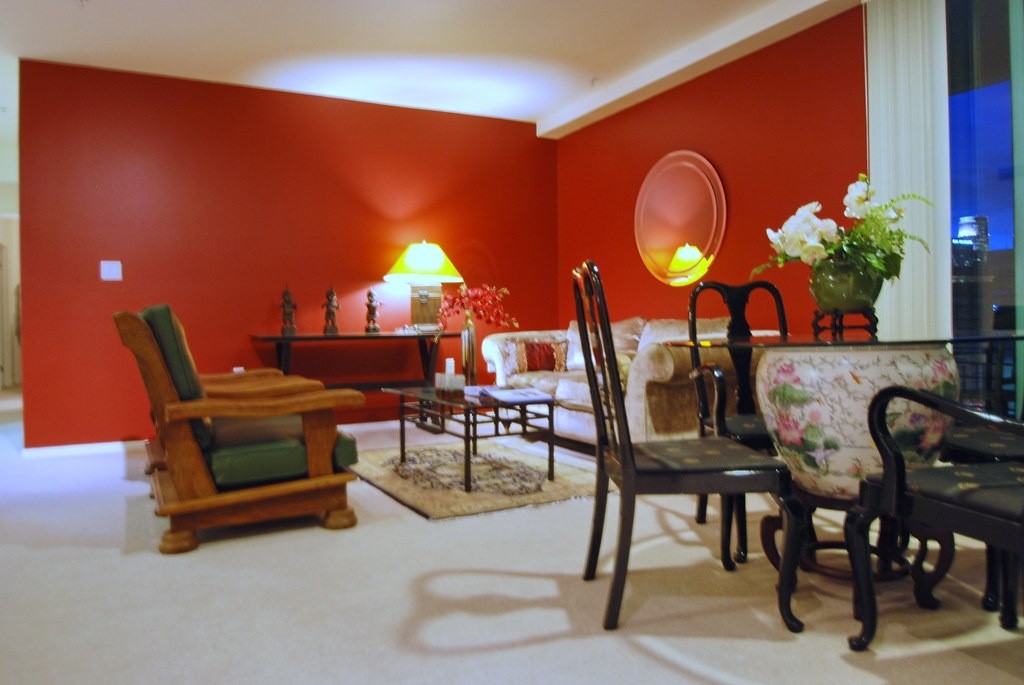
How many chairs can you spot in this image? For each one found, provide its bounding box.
[112,304,365,555]
[897,306,1024,612]
[687,280,819,572]
[571,258,806,634]
[843,387,1024,652]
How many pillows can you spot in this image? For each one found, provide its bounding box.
[638,316,731,352]
[566,316,647,370]
[505,339,569,377]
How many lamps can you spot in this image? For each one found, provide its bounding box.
[383,239,465,326]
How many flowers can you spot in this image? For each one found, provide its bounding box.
[440,283,520,330]
[749,173,935,286]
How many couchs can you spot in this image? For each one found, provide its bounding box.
[481,330,792,445]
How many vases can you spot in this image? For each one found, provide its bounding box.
[808,258,883,312]
[459,326,477,385]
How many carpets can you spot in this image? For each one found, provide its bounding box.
[350,440,618,522]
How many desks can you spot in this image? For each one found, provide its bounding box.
[380,386,558,493]
[654,332,1024,652]
[249,331,461,423]
